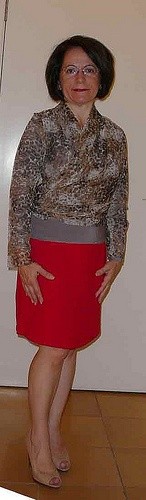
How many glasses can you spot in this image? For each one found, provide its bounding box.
[60,64,100,77]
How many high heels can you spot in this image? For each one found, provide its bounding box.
[52,447,71,471]
[26,428,62,488]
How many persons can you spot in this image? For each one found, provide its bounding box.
[7,35,129,487]
